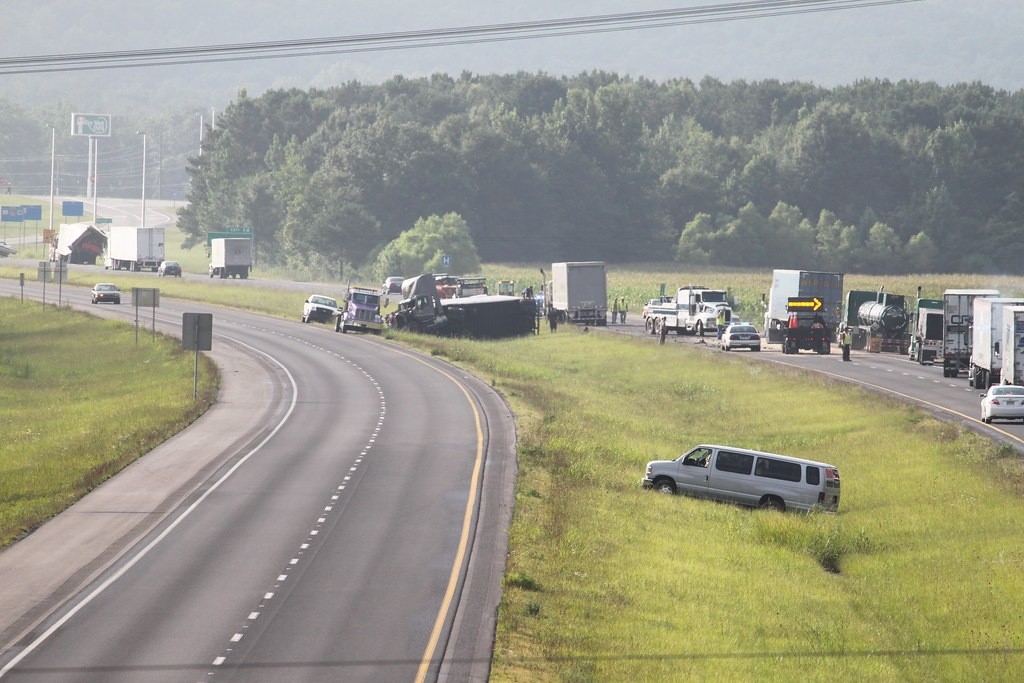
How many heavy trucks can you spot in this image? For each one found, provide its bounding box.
[642,286,740,337]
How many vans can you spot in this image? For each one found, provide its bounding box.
[640,443,841,517]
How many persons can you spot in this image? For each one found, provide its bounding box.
[546,307,559,333]
[523,285,534,301]
[618,297,628,324]
[658,317,666,344]
[610,298,619,323]
[716,308,724,340]
[701,450,712,467]
[843,327,852,361]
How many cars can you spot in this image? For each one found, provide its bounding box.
[978,385,1024,424]
[91,283,121,304]
[382,276,404,294]
[157,261,182,278]
[721,322,761,351]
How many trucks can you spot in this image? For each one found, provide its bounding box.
[761,269,844,344]
[333,280,383,336]
[545,261,608,326]
[57,221,254,279]
[910,269,1024,391]
[835,290,909,355]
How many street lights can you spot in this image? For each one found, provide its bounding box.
[137,130,147,228]
[46,123,57,227]
[193,110,202,171]
[211,105,215,131]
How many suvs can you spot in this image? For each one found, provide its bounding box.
[301,295,338,323]
[781,316,830,356]
[435,275,459,299]
